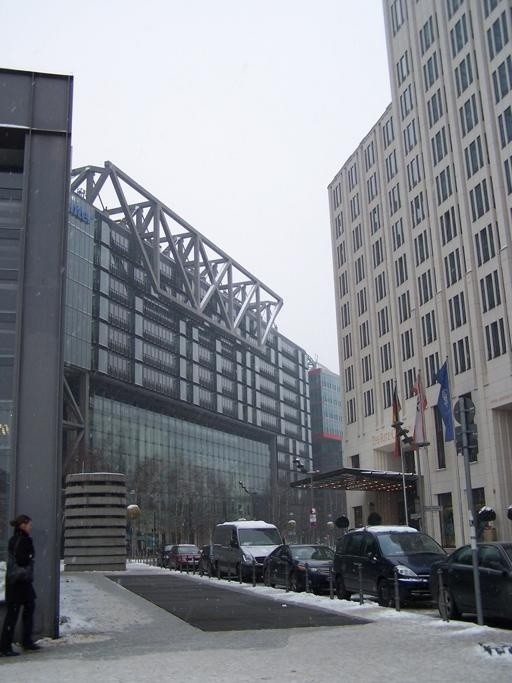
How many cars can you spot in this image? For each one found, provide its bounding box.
[157,544,210,575]
[263,525,512,619]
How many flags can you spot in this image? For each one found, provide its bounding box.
[436,359,456,442]
[392,387,403,458]
[413,375,428,448]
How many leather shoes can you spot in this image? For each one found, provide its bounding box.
[0,643,39,656]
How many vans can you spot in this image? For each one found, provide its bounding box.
[210,521,284,582]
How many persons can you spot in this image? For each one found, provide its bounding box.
[0,514,43,657]
